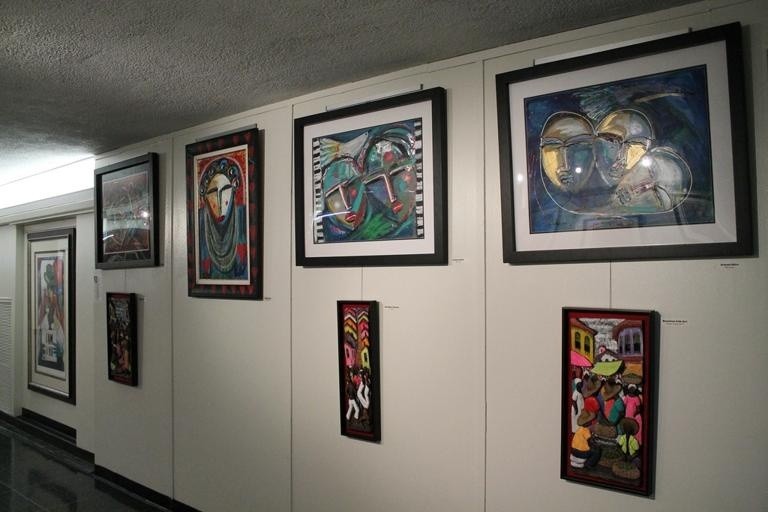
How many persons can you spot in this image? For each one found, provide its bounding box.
[571,345,643,471]
[191,157,248,281]
[346,369,371,421]
[320,120,418,240]
[541,111,692,212]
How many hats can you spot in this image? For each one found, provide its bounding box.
[623,374,642,384]
[603,381,622,401]
[577,408,596,426]
[581,378,601,398]
[620,417,640,436]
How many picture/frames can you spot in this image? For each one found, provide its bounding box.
[336,300,382,443]
[495,20,756,264]
[184,126,266,300]
[27,227,76,405]
[559,307,662,500]
[105,292,139,387]
[93,150,162,271]
[294,87,447,269]
